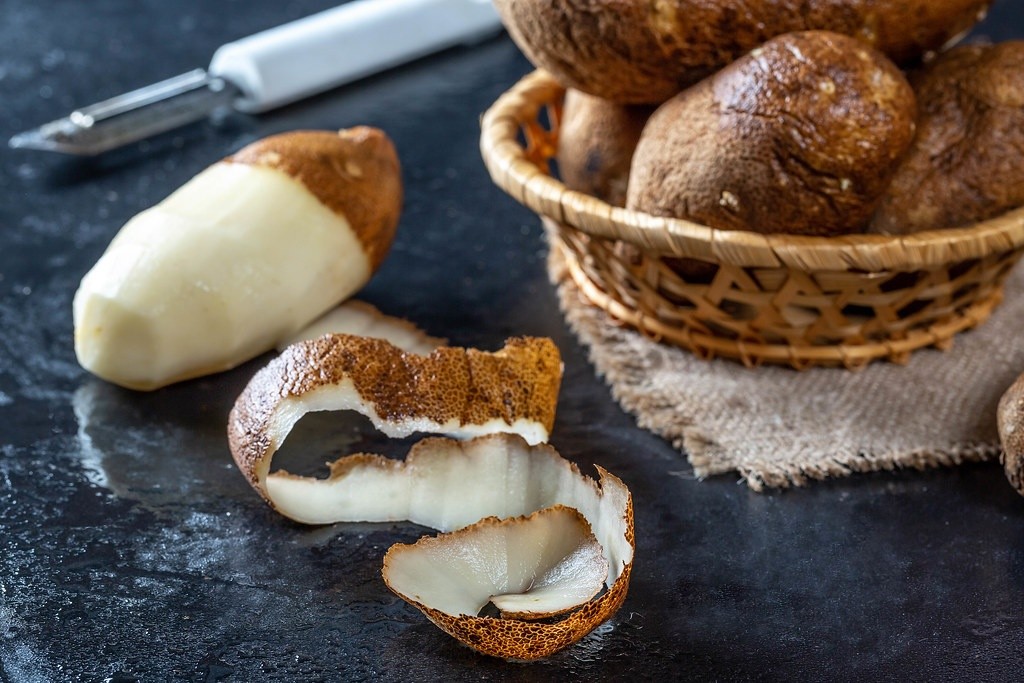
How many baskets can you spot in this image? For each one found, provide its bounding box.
[479,65,1023,370]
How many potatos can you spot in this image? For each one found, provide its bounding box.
[72,125,398,389]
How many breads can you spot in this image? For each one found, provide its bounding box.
[497,1,1024,300]
